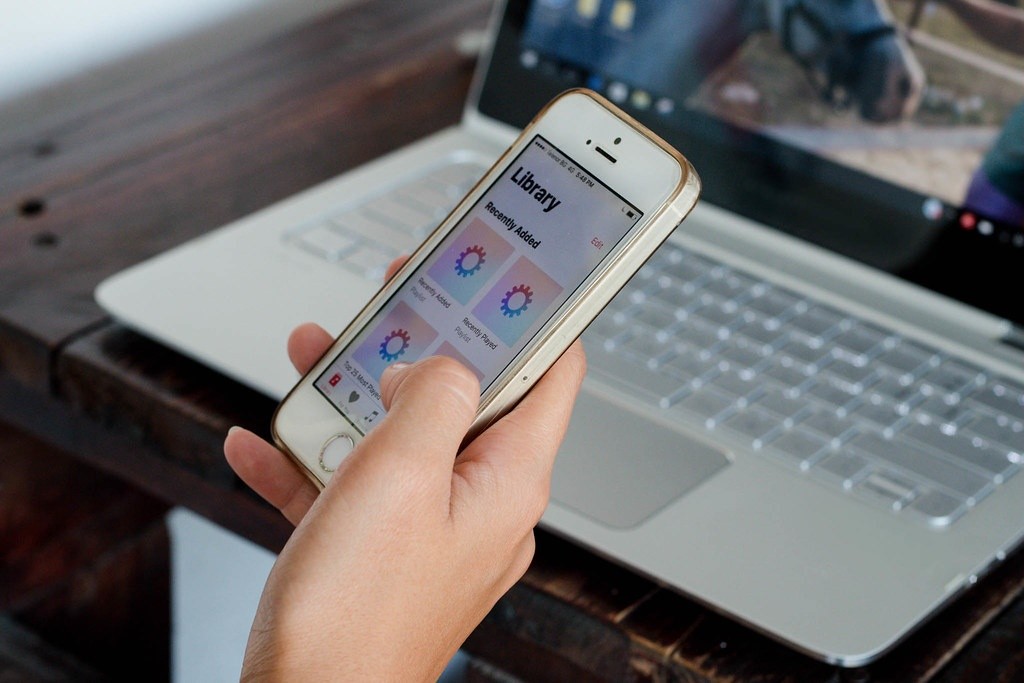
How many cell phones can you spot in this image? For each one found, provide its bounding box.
[271,86,704,497]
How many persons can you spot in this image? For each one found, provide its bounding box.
[223,253,588,683]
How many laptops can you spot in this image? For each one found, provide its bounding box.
[90,0,1024,668]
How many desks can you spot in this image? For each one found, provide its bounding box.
[0,0,1024,683]
